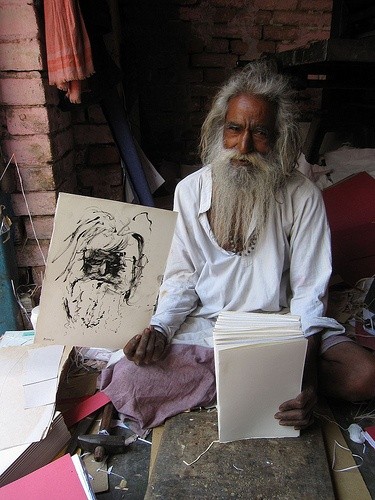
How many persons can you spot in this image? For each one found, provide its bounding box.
[105,56,375,431]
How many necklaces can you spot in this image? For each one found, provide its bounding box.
[226,225,260,256]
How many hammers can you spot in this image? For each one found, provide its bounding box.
[75,401,127,463]
